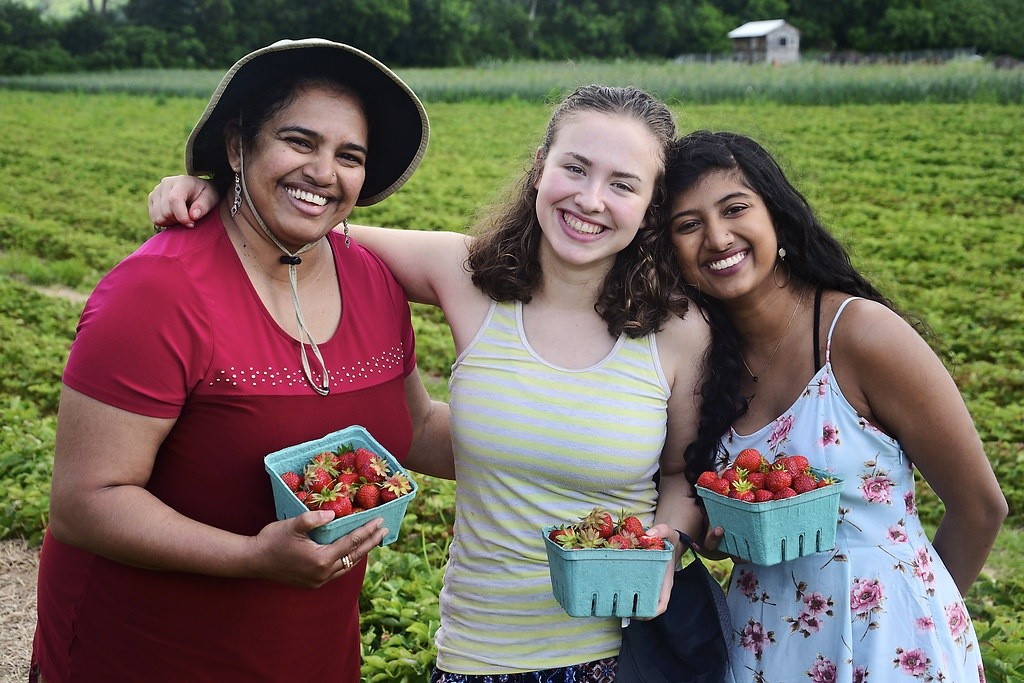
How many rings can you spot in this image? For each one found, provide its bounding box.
[341,554,353,569]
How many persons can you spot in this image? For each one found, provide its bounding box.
[31,38,457,683]
[665,131,1008,683]
[146,84,714,683]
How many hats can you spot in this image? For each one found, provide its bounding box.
[183,38,430,206]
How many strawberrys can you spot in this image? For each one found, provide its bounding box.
[547,505,665,550]
[280,441,413,520]
[696,449,839,502]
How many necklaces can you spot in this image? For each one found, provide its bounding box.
[739,282,806,383]
